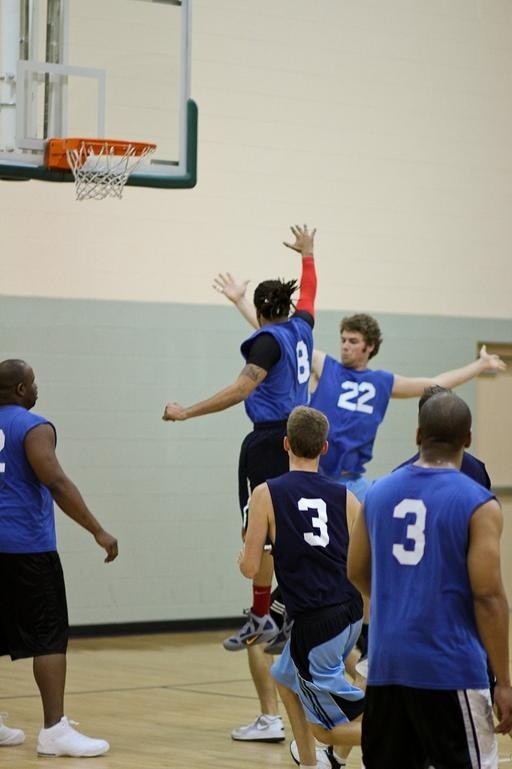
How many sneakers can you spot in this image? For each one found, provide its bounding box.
[230,713,285,742]
[290,739,346,769]
[37,716,110,758]
[223,611,294,654]
[0,722,25,745]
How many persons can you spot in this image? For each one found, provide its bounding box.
[238,405,368,769]
[165,222,319,654]
[1,359,119,759]
[290,384,491,769]
[346,391,512,769]
[230,583,288,740]
[209,273,507,500]
